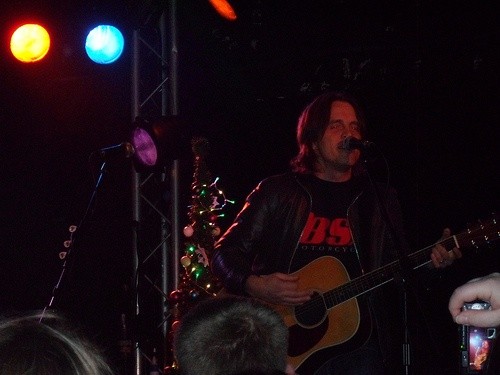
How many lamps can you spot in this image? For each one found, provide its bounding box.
[129,113,192,173]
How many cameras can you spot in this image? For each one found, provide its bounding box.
[459,303,500,375]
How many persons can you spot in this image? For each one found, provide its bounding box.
[211,92,462,375]
[175,293,289,375]
[0,310,116,375]
[448,272,500,328]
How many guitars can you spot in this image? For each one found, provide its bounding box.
[247,212,500,375]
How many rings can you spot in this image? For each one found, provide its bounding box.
[439,257,445,265]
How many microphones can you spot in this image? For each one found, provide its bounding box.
[92,141,135,160]
[343,136,377,149]
